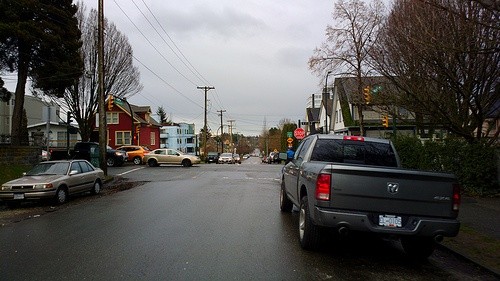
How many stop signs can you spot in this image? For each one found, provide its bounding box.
[293,128,306,140]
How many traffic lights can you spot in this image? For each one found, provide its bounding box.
[108,95,114,110]
[364,86,371,105]
[382,113,389,127]
[67,111,73,124]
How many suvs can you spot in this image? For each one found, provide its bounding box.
[72,141,127,168]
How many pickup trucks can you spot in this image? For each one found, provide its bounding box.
[278,134,461,261]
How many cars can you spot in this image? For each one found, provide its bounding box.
[243,151,278,164]
[115,145,150,165]
[218,152,236,164]
[0,159,106,208]
[206,152,220,164]
[232,153,241,164]
[140,149,201,167]
